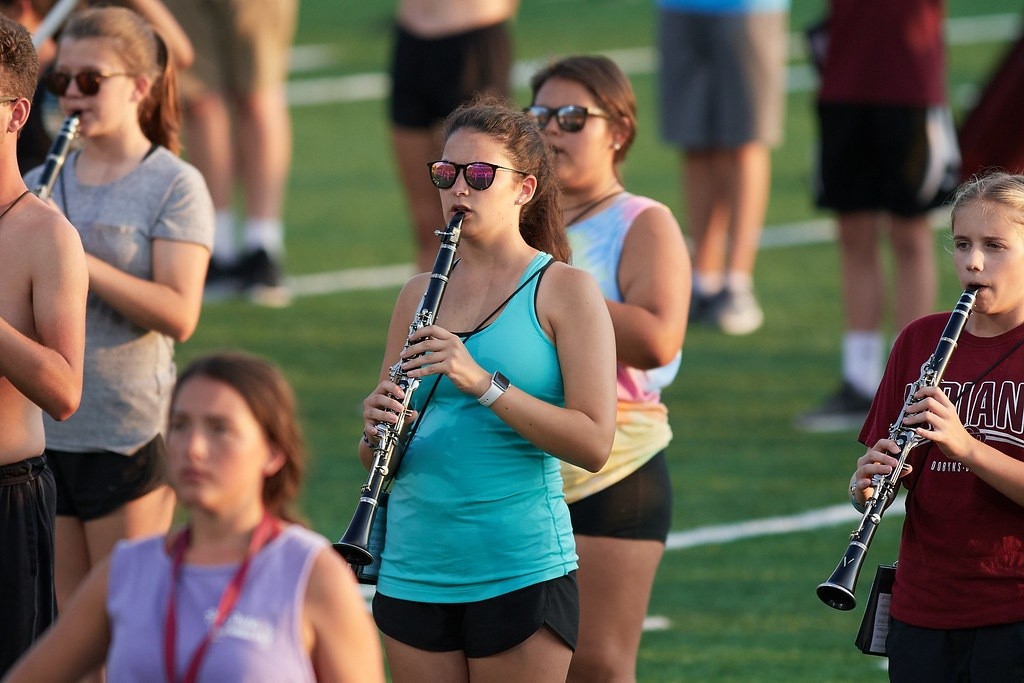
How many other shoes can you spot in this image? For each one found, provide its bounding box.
[693,284,764,334]
[204,245,295,308]
[838,379,875,409]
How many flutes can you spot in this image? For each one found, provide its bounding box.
[814,284,985,614]
[331,209,466,568]
[34,108,82,200]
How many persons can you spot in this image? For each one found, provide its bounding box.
[522,53,692,683]
[654,1,787,337]
[358,98,618,683]
[384,0,515,277]
[0,0,383,683]
[849,174,1024,683]
[796,0,962,434]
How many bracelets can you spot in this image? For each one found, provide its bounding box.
[361,427,377,449]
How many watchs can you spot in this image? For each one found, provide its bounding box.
[476,371,513,408]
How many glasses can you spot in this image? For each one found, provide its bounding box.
[522,106,613,135]
[46,67,137,97]
[426,160,526,194]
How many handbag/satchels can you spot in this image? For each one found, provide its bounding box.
[353,490,388,585]
[855,558,899,657]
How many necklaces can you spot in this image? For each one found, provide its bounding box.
[0,189,31,219]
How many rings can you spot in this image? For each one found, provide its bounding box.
[850,483,856,493]
